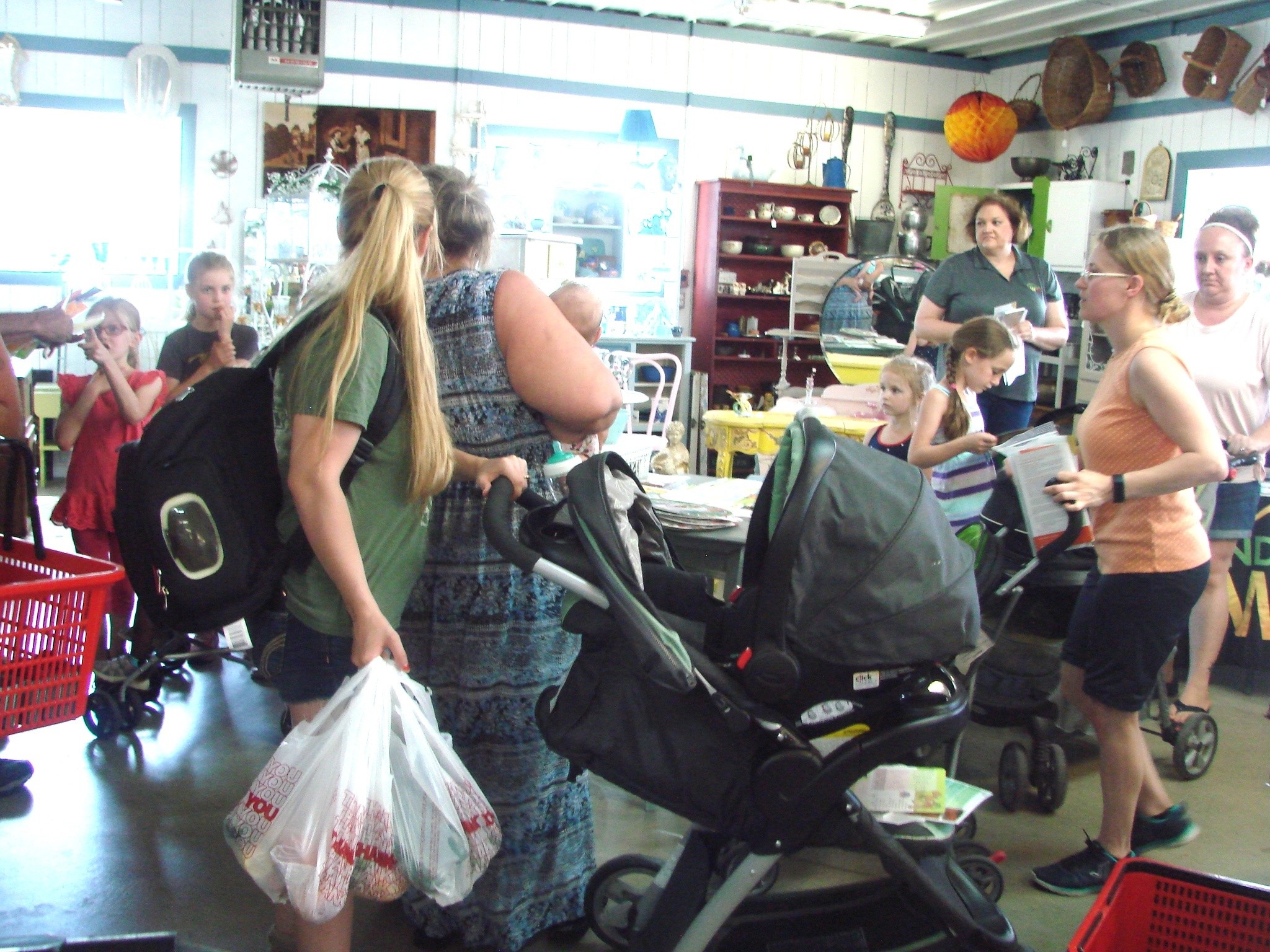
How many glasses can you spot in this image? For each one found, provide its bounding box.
[85,325,136,337]
[1079,266,1131,279]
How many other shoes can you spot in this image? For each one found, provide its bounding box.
[95,656,151,690]
[1151,668,1181,701]
[0,757,33,794]
[189,630,220,665]
[1165,699,1210,730]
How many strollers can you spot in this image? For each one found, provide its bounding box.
[926,471,1083,808]
[1146,657,1221,780]
[79,616,307,742]
[482,414,1024,952]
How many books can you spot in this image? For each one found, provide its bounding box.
[1006,433,1097,557]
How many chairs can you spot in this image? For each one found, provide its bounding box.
[612,350,686,441]
[763,250,863,392]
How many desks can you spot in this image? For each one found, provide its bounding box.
[643,470,752,602]
[700,408,887,481]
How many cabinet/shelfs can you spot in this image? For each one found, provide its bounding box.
[928,170,1128,276]
[596,333,697,444]
[693,177,860,402]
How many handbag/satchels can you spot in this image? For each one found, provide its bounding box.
[222,656,505,923]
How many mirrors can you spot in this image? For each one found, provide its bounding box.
[814,254,940,387]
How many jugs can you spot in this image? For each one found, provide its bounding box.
[822,156,851,189]
[756,202,775,220]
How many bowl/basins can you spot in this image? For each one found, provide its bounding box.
[780,244,804,258]
[640,366,675,382]
[719,240,743,254]
[772,205,796,220]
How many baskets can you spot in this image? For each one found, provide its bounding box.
[1,438,127,733]
[1066,857,1270,952]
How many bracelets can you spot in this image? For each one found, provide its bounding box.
[1113,474,1124,505]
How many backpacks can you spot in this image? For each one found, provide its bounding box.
[117,293,408,638]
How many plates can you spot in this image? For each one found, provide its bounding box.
[819,205,841,225]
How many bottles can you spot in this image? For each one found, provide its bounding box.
[738,315,745,337]
[724,321,741,337]
[542,440,583,520]
[746,316,758,333]
[671,326,683,337]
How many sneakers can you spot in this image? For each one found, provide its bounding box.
[1029,841,1137,897]
[1132,800,1199,858]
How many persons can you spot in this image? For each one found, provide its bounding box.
[248,156,533,951]
[863,354,939,485]
[548,283,609,456]
[1029,225,1196,899]
[157,251,259,668]
[0,334,33,795]
[1148,207,1270,728]
[330,122,373,167]
[906,315,1016,537]
[0,298,73,344]
[55,296,167,702]
[400,165,624,949]
[652,421,690,476]
[917,195,1069,432]
[852,260,877,306]
[819,256,883,334]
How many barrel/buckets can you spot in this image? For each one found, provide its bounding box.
[853,219,895,260]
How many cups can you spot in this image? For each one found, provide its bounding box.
[797,213,814,223]
